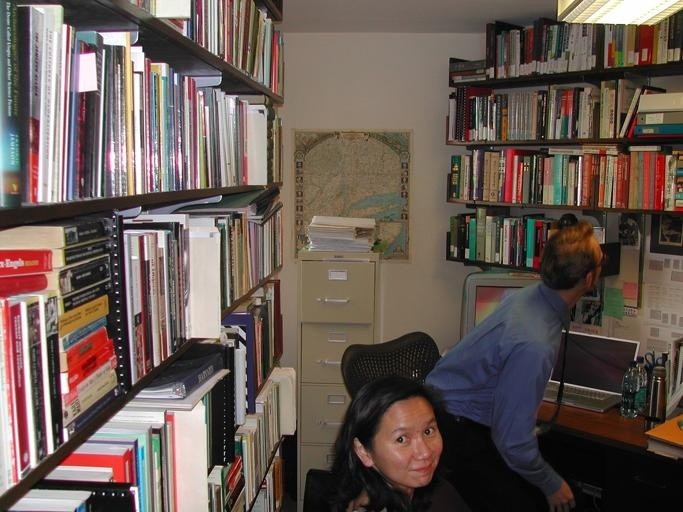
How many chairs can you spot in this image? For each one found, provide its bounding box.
[340,330,440,400]
[302,468,334,512]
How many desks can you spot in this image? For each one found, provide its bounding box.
[535,400,682,512]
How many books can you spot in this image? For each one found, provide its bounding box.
[645,335,683,460]
[306,215,379,254]
[0,1,296,512]
[444,10,683,271]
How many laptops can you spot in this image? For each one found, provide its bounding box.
[543,328,644,414]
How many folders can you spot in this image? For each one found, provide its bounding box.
[134,351,225,401]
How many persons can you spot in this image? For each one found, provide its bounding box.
[318,371,471,510]
[422,220,606,512]
[583,301,603,327]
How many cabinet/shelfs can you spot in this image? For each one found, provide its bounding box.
[295,246,382,512]
[0,0,284,512]
[444,55,682,278]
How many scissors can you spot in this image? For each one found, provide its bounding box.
[645,352,663,368]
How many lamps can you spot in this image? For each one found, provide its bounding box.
[556,0,682,29]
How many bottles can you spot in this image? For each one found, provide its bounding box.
[621,361,641,417]
[634,355,647,411]
[646,366,667,430]
